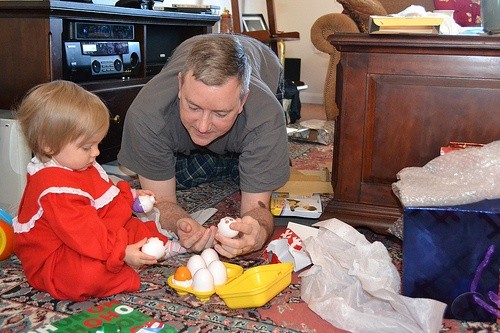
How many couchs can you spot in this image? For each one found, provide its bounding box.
[311,0,435,121]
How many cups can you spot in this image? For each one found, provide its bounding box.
[433,10,454,18]
[481,0,500,34]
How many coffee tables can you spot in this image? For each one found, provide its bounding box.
[318,33,500,234]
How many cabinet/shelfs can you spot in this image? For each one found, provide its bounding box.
[0,0,220,165]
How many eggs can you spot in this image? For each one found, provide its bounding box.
[172,248,227,293]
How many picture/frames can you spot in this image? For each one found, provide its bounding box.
[242,14,269,33]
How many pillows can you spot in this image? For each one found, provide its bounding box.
[336,0,387,33]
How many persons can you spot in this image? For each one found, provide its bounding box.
[12,81,172,302]
[117,34,289,259]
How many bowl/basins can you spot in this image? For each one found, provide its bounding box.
[167,259,294,309]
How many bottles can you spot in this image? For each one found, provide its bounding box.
[220,8,232,34]
[210,5,221,34]
